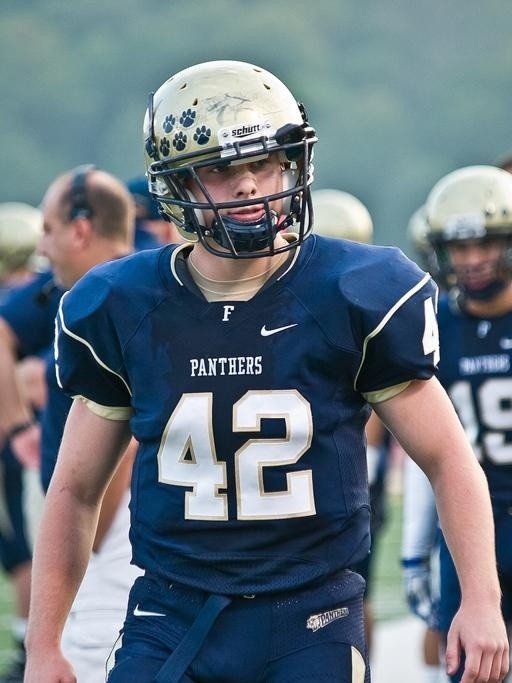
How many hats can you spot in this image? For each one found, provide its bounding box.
[124,173,163,222]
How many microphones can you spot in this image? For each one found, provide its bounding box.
[36,282,56,303]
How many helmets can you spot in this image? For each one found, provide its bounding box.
[423,163,512,302]
[286,188,374,246]
[405,202,440,275]
[141,58,319,260]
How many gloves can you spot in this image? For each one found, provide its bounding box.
[398,551,442,633]
